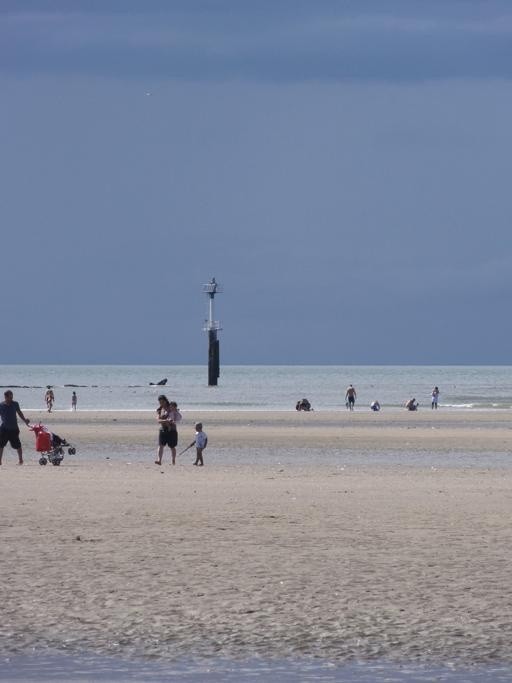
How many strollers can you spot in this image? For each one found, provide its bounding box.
[26,421,76,466]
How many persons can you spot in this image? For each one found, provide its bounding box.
[0,389,31,467]
[344,383,358,412]
[162,401,182,432]
[405,398,420,411]
[71,390,77,412]
[154,394,183,466]
[431,386,440,411]
[370,400,381,412]
[44,384,55,413]
[191,422,208,466]
